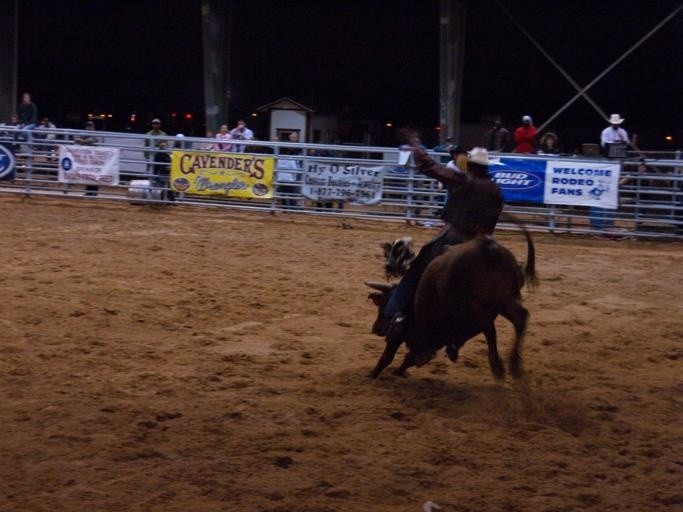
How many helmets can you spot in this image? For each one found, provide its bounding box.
[449,144,463,152]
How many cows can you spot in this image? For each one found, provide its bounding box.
[363,210,539,387]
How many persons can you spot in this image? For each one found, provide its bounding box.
[540,133,561,152]
[513,114,537,153]
[281,131,300,206]
[15,92,37,135]
[377,138,504,343]
[36,117,56,146]
[396,130,427,228]
[74,121,99,195]
[433,135,465,164]
[484,119,512,154]
[600,114,629,157]
[4,114,19,126]
[140,113,258,195]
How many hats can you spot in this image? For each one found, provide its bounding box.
[607,113,625,125]
[539,132,558,145]
[465,147,499,166]
